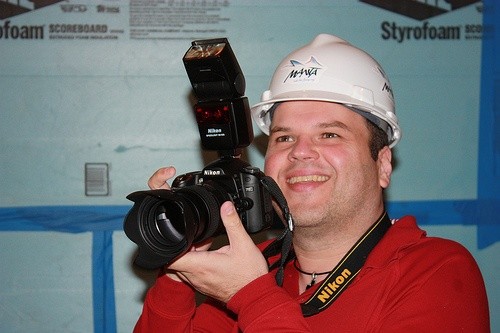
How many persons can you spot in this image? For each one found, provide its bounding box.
[132,33,490,333]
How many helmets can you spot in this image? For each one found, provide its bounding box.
[250,33,402,149]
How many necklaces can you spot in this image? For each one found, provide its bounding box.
[293,257,333,291]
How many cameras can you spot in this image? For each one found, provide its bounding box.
[123,38,274,274]
